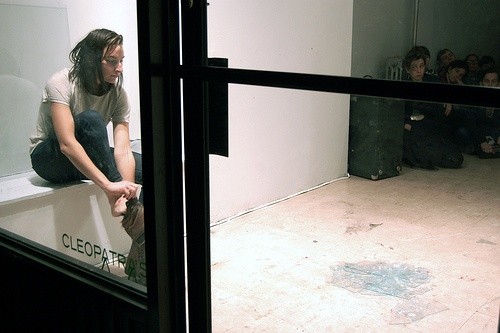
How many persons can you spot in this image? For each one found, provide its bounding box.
[400,43,500,165]
[30,28,148,285]
